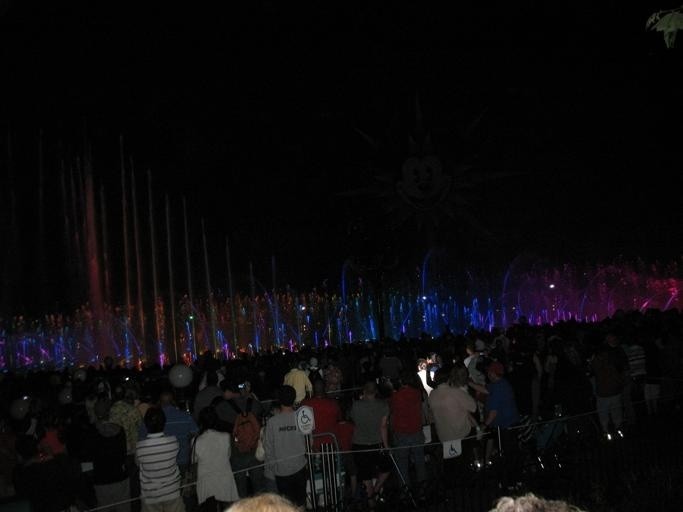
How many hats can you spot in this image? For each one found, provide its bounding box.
[486,361,504,374]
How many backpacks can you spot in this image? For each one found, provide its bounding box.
[234,411,261,454]
